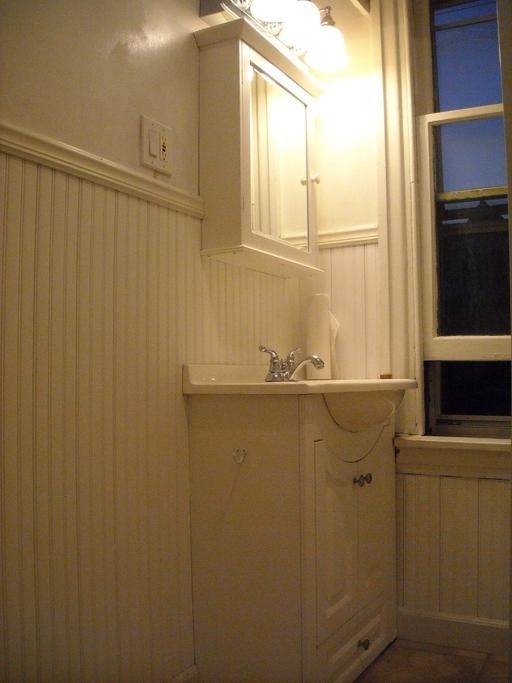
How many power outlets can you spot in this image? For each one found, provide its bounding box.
[141,114,174,174]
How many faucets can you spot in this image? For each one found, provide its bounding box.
[281,355,325,380]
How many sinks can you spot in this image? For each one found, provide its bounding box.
[322,379,406,433]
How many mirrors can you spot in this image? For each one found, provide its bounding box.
[238,40,318,261]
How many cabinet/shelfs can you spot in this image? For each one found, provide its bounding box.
[311,423,395,683]
